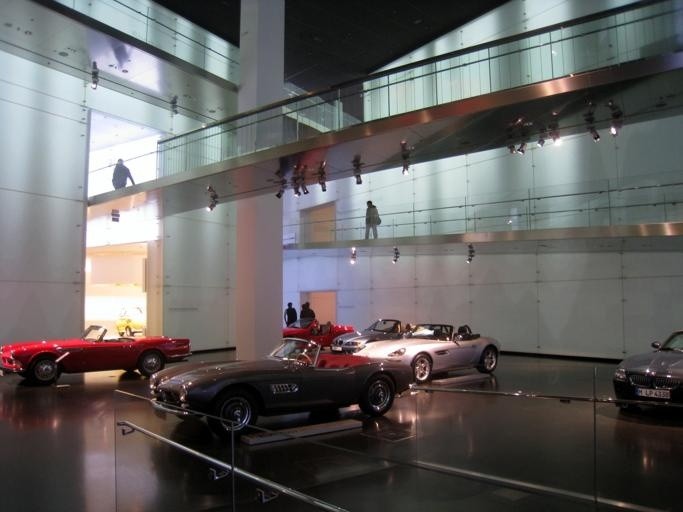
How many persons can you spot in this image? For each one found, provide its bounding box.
[112,159,135,190]
[365,201,379,239]
[300,302,315,327]
[284,302,297,327]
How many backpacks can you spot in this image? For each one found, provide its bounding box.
[376,216,381,224]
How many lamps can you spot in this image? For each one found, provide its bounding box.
[168,97,179,115]
[504,103,683,155]
[91,69,100,89]
[464,245,476,263]
[206,142,416,211]
[392,246,400,265]
[350,249,358,263]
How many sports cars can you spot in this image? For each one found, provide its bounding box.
[613,331,683,417]
[0,325,191,384]
[329,319,423,352]
[283,317,353,348]
[149,337,412,438]
[352,323,502,384]
